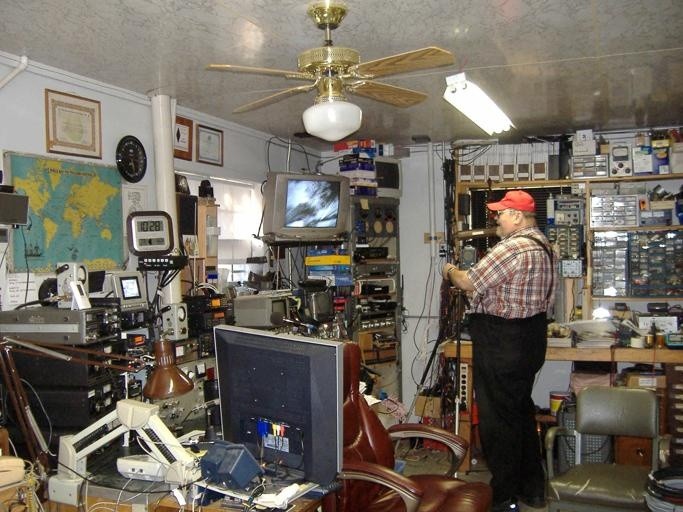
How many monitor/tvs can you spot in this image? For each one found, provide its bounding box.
[373,156,403,199]
[263,171,351,242]
[111,271,149,313]
[213,323,344,489]
[233,295,291,328]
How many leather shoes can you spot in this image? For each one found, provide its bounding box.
[518,496,549,512]
[494,497,518,512]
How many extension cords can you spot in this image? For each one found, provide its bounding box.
[273,271,281,290]
[459,362,469,412]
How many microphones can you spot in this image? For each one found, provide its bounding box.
[270,311,315,330]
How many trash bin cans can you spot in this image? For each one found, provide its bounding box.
[646,468,682,512]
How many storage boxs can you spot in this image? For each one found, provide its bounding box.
[614,435,651,467]
[414,390,443,419]
[654,389,667,435]
[423,414,453,451]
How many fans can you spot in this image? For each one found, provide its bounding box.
[206,0,455,113]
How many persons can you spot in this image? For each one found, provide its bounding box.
[442,190,557,512]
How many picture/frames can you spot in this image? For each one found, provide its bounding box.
[196,123,224,167]
[45,88,102,159]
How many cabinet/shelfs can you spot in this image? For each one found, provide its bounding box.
[454,171,683,322]
[347,195,400,364]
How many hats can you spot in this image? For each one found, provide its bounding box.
[484,189,537,212]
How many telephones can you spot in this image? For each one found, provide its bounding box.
[0,456,25,487]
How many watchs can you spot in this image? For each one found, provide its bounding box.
[447,267,456,281]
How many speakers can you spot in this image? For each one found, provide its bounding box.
[216,443,267,492]
[199,439,234,484]
[161,302,189,341]
[307,291,331,323]
[57,262,89,309]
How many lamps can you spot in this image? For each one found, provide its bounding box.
[441,72,518,137]
[0,329,195,503]
[302,90,362,142]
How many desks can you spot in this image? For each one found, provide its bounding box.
[443,338,683,472]
[0,481,320,511]
[644,467,683,512]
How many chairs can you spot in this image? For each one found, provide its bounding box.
[543,384,662,512]
[320,342,495,512]
